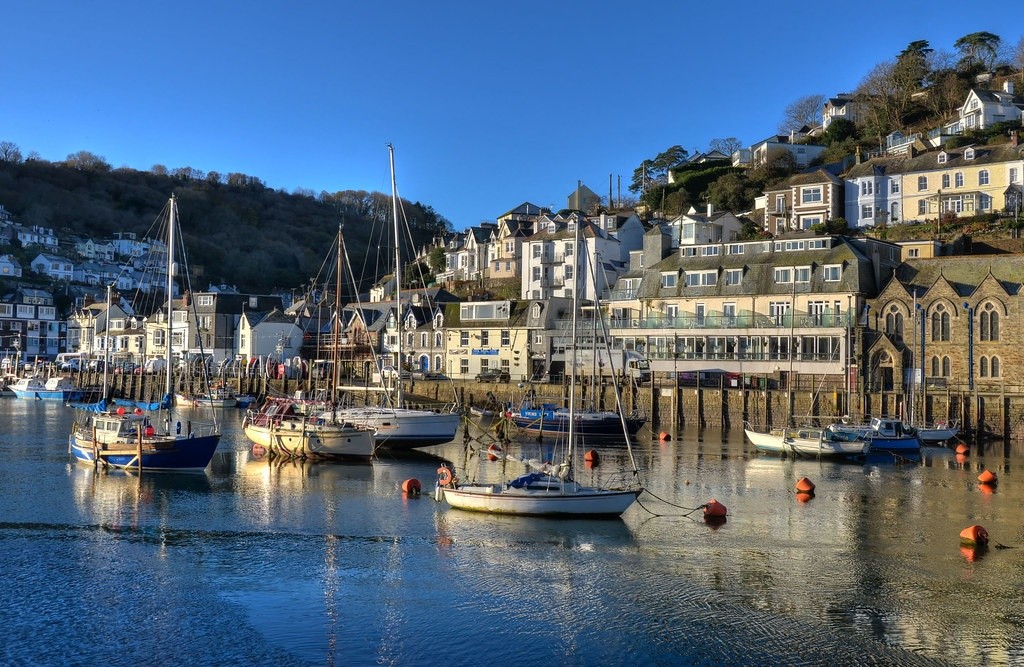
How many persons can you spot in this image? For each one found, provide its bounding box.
[800,417,809,439]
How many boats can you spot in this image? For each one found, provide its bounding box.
[0,372,23,399]
[233,389,258,409]
[172,390,238,408]
[5,373,87,403]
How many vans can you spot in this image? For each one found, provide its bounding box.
[0,355,24,372]
[54,353,81,365]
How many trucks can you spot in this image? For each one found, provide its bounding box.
[61,357,88,374]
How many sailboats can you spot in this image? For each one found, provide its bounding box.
[742,296,873,458]
[822,289,921,452]
[899,412,960,446]
[241,219,377,467]
[439,180,644,518]
[314,140,462,453]
[507,250,648,437]
[68,192,223,481]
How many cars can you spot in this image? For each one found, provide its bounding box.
[84,357,147,376]
[411,368,431,381]
[380,365,412,380]
[474,368,511,384]
[21,362,43,372]
